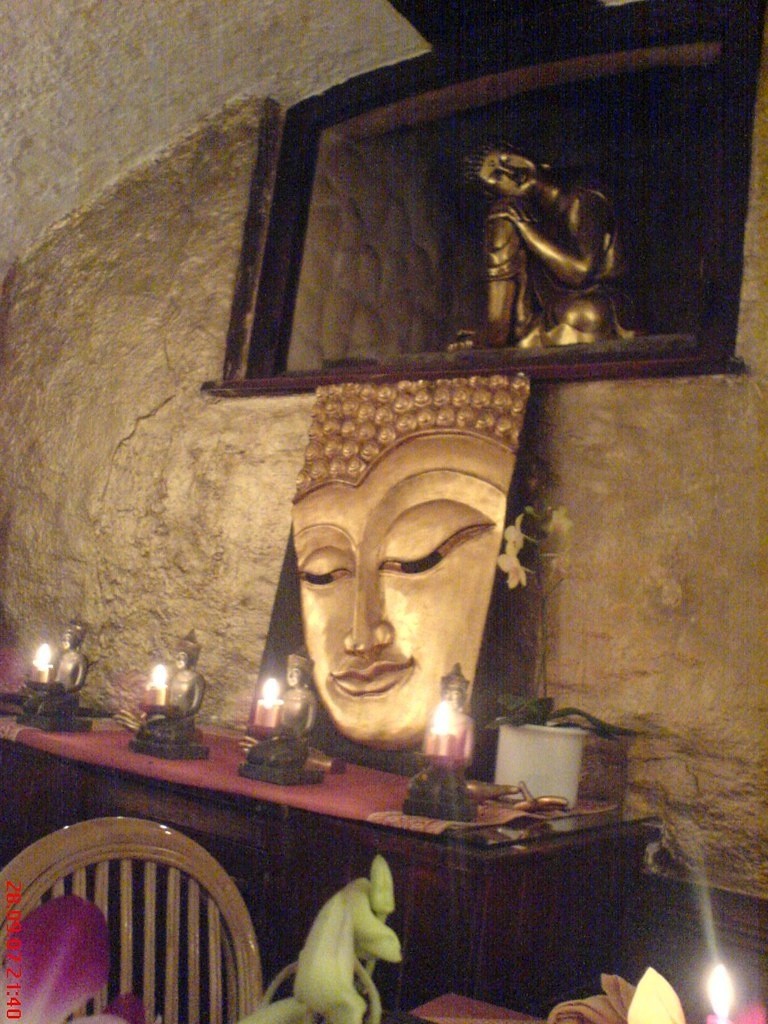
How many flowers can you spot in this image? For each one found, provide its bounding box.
[0,895,145,1024]
[486,499,643,741]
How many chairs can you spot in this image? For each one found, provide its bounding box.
[0,817,263,1024]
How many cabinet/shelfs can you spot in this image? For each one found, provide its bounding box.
[0,716,659,1024]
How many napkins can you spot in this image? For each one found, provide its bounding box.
[549,967,686,1023]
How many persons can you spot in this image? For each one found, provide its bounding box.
[13,610,91,734]
[127,628,211,760]
[399,661,482,825]
[291,369,530,749]
[445,133,657,353]
[235,644,324,785]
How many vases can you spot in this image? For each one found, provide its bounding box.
[493,718,589,810]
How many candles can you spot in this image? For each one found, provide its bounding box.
[145,681,170,707]
[426,728,457,756]
[32,664,53,683]
[253,699,283,727]
